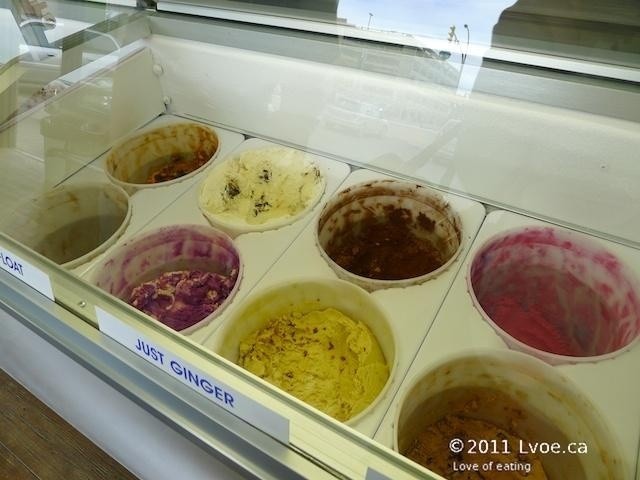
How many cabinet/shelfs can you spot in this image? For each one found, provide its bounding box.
[0,0,639,480]
